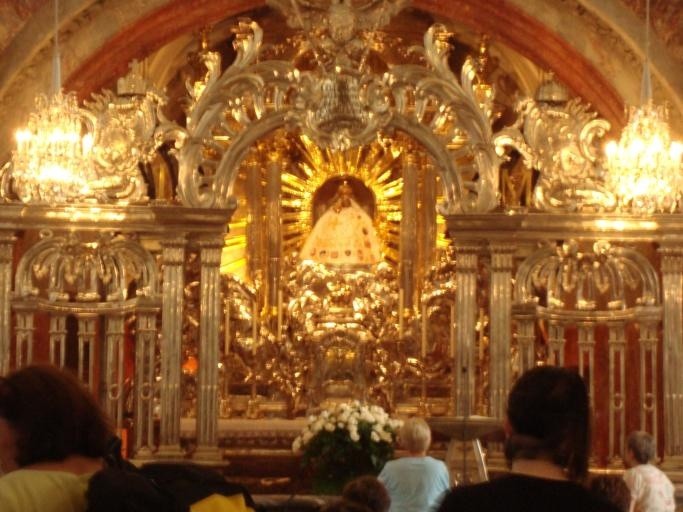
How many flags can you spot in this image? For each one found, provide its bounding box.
[312,466,361,495]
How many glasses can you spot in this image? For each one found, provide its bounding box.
[600,0,683,216]
[11,0,95,208]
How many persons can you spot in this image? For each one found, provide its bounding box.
[0,360,131,512]
[340,476,391,511]
[589,471,632,511]
[434,363,623,511]
[375,415,454,512]
[619,430,678,511]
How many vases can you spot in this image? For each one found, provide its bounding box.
[294,400,404,482]
